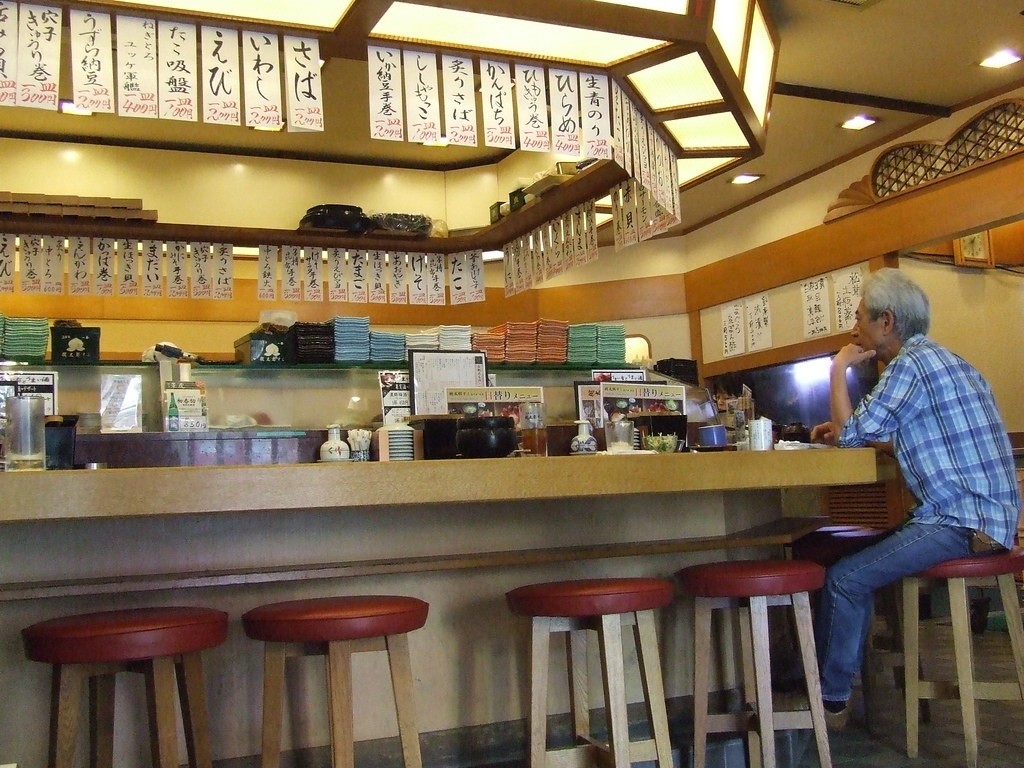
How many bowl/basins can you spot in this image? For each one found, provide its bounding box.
[455,416,516,459]
[299,204,372,237]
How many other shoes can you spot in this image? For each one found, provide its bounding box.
[773,694,848,730]
[772,670,804,691]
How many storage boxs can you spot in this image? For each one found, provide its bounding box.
[50,327,101,360]
[490,201,507,225]
[234,332,295,364]
[509,187,526,212]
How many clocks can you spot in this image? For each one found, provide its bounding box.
[953,229,995,269]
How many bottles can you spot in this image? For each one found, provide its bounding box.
[319,424,350,461]
[784,422,811,443]
[169,392,179,431]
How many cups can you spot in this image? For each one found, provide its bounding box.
[519,402,548,457]
[748,419,773,451]
[772,424,787,444]
[604,421,634,454]
[3,396,47,472]
[696,425,727,446]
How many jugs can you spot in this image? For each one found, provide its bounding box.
[571,420,597,454]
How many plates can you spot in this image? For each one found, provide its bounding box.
[376,423,415,461]
[689,446,737,452]
[0,312,50,364]
[288,317,626,367]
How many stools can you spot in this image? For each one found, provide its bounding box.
[672,559,832,768]
[901,544,1024,768]
[240,595,431,768]
[505,577,675,768]
[21,605,230,768]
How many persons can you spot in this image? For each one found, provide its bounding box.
[749,267,1021,730]
[716,372,743,410]
